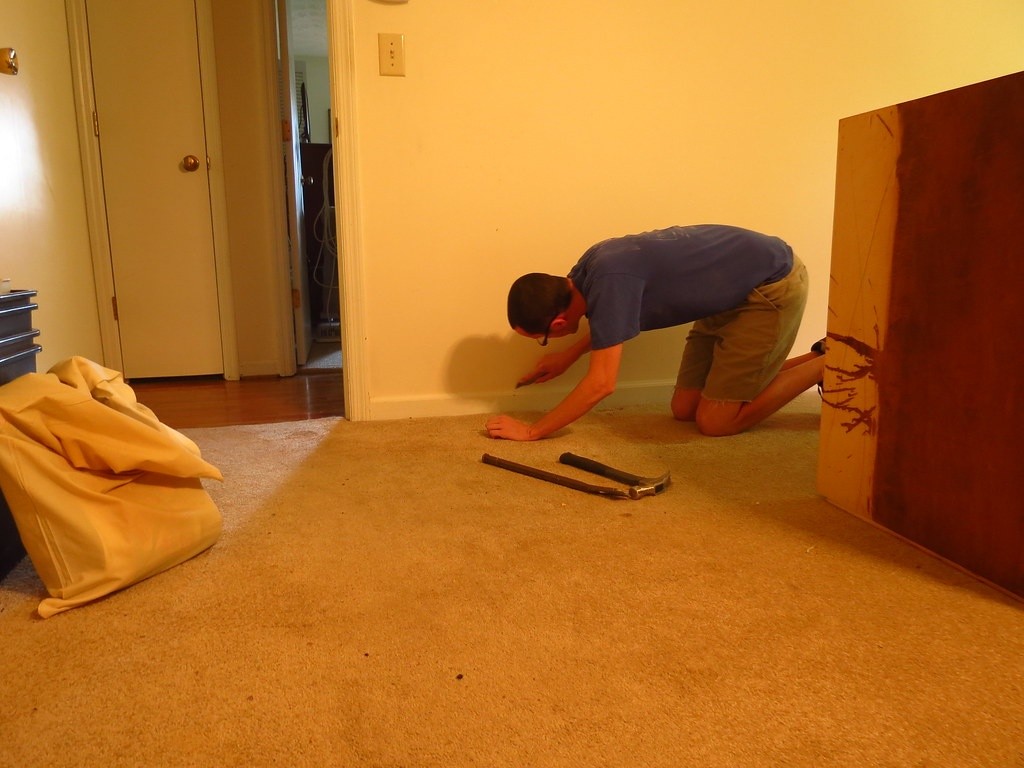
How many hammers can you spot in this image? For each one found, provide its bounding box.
[558,452,673,500]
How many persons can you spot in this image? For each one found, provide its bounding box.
[485,224,827,442]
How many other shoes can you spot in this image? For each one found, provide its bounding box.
[811,335,828,396]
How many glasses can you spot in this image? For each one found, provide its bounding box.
[535,312,560,347]
